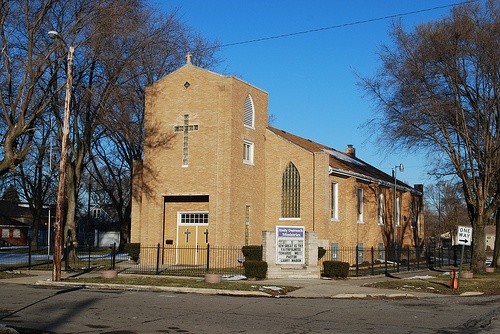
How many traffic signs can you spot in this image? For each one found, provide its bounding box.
[456,224,473,246]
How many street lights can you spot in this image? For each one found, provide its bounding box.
[392,163,404,272]
[48,29,76,283]
